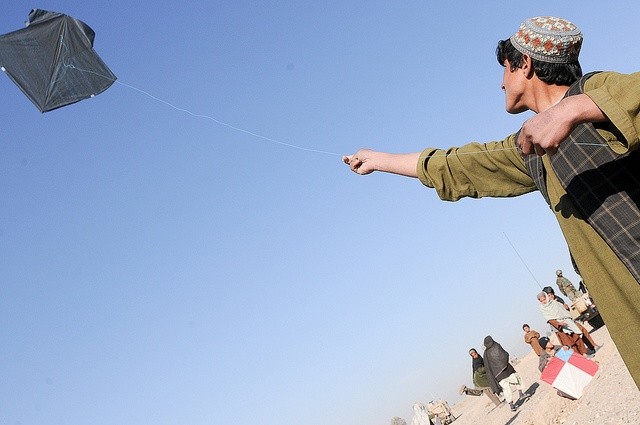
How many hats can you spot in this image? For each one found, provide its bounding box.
[509,14,585,64]
[539,337,549,349]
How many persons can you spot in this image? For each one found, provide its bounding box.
[537,292,595,358]
[482,335,531,411]
[542,286,599,354]
[340,14,640,390]
[459,348,502,406]
[0,7,118,113]
[523,324,543,356]
[555,270,579,303]
[538,337,570,372]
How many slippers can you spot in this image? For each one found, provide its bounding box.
[512,405,518,411]
[520,393,531,401]
[459,385,466,395]
